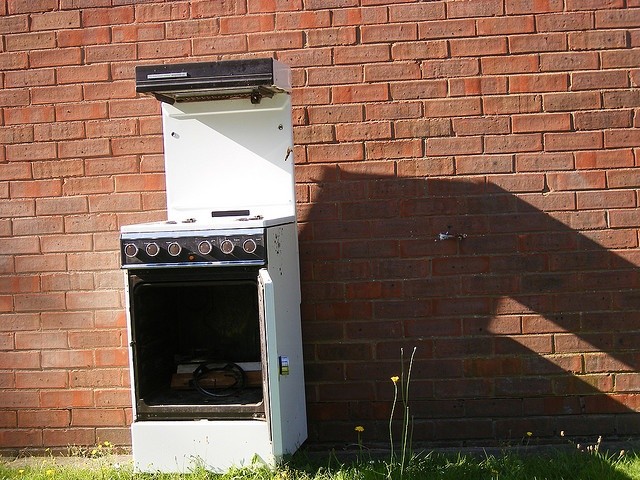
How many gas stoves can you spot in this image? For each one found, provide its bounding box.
[119,215,296,269]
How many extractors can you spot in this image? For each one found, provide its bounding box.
[131,59,292,103]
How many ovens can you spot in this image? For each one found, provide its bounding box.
[119,267,309,476]
